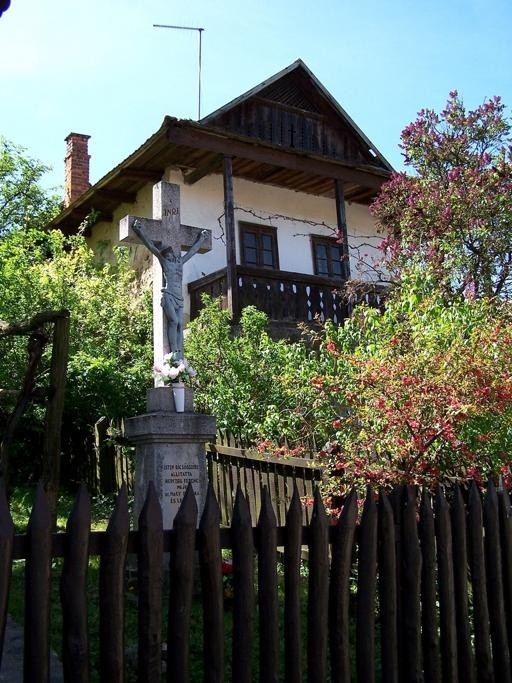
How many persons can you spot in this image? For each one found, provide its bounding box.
[132,219,208,368]
